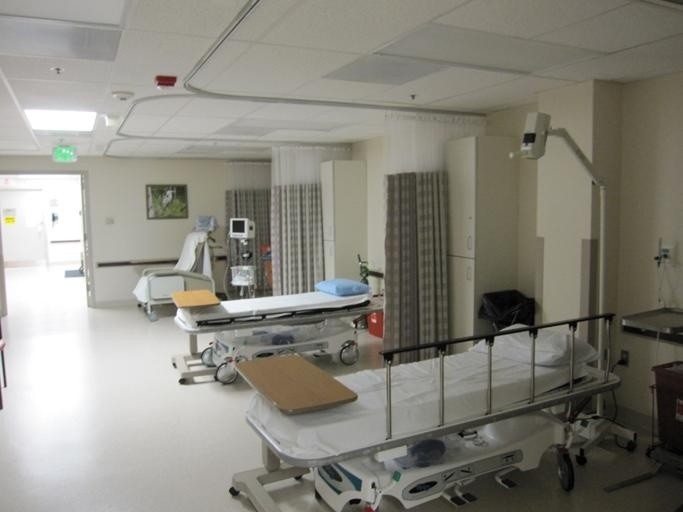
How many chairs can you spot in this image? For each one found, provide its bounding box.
[136,228,216,321]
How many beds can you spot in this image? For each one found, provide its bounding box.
[169,287,384,386]
[229,324,637,512]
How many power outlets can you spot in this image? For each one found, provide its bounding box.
[620,350,627,366]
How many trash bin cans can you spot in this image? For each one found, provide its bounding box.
[367,294,383,337]
[652,360,683,454]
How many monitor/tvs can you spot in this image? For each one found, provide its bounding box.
[230,218,255,238]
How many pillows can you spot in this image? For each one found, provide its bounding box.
[468,321,589,367]
[315,278,369,295]
